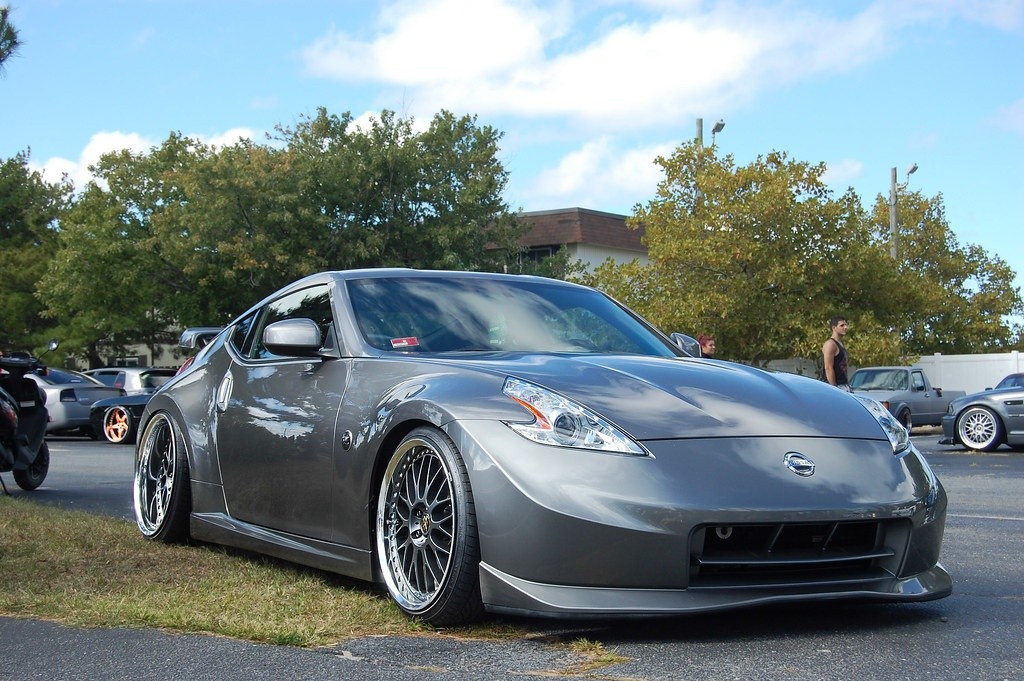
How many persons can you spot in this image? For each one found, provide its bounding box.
[821,316,854,394]
[698,334,715,360]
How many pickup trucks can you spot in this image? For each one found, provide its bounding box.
[840,364,966,436]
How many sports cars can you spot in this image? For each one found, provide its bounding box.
[132,266,956,631]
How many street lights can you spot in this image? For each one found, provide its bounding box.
[696,116,725,202]
[890,163,919,260]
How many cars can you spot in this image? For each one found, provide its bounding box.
[25,363,128,437]
[941,372,1024,452]
[89,393,158,444]
[81,365,181,397]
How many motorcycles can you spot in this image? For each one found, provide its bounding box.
[0,336,61,495]
